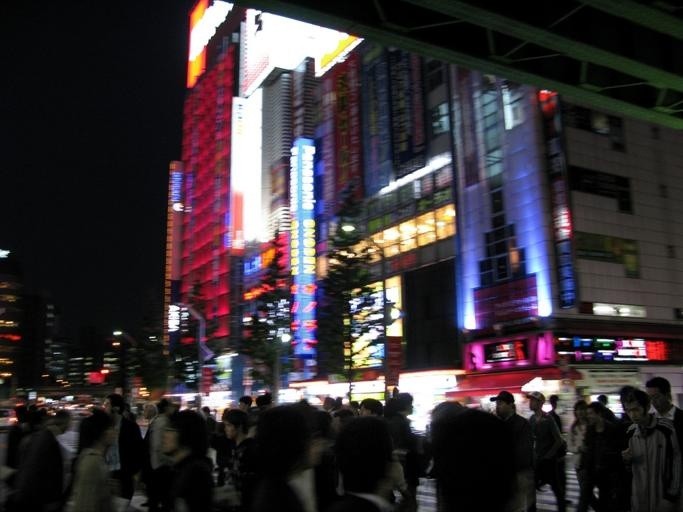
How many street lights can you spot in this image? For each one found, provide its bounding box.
[341,222,387,404]
[168,302,205,418]
[113,328,142,418]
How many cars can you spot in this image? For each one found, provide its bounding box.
[0,399,106,429]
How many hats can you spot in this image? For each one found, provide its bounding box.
[490,391,515,405]
[526,390,545,404]
